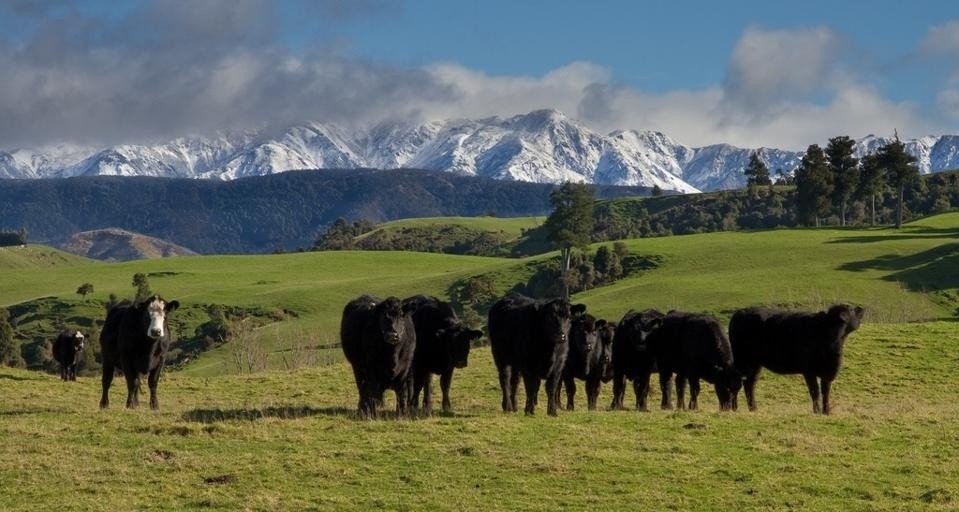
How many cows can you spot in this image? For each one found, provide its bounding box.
[98,294,180,410]
[488,289,742,417]
[728,302,867,415]
[52,330,90,382]
[340,293,484,422]
